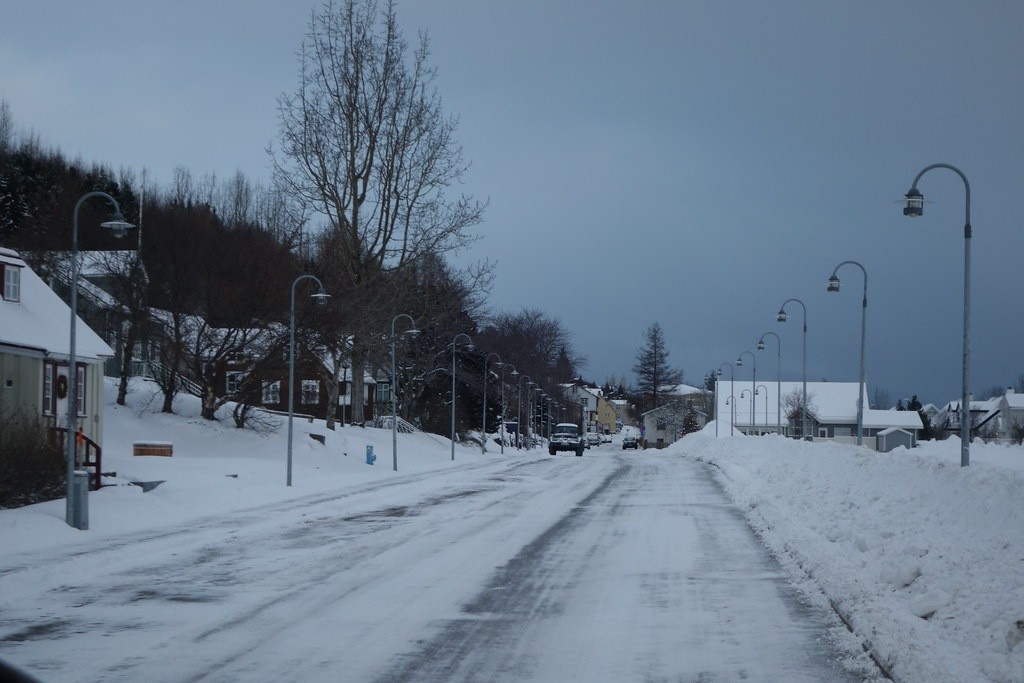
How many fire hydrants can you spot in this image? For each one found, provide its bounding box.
[366,444,377,466]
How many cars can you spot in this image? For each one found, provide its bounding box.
[621,437,638,449]
[616,421,624,430]
[587,433,600,447]
[601,433,613,444]
[583,437,590,449]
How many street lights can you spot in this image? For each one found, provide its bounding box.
[826,260,868,446]
[903,162,974,466]
[776,298,808,441]
[283,272,330,484]
[481,352,566,455]
[66,185,137,533]
[390,310,422,471]
[448,333,475,461]
[756,331,782,436]
[716,351,769,436]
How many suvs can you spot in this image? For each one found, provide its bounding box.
[549,423,583,455]
